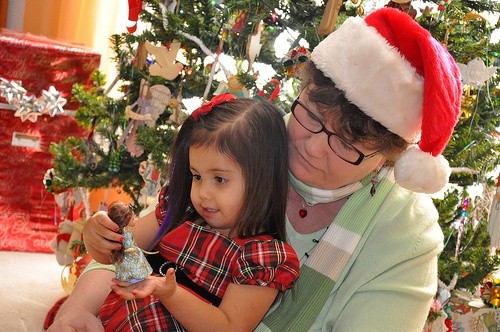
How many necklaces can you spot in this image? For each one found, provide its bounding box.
[297,195,310,218]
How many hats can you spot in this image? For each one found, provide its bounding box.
[310,7,464,194]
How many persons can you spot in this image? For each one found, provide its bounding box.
[81,92,299,332]
[107,201,152,285]
[46,7,461,331]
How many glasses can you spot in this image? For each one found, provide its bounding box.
[289,84,383,166]
